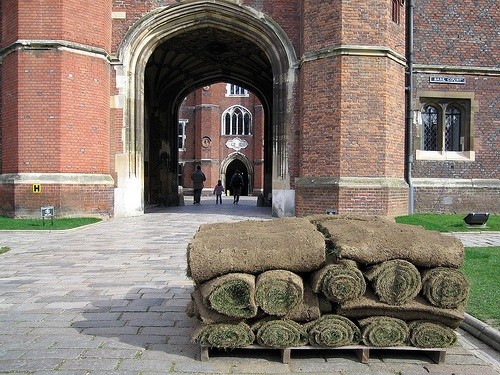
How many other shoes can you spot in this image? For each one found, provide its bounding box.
[216,202,222,204]
[233,201,238,205]
[194,202,200,205]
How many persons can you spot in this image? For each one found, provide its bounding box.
[191,166,206,205]
[229,170,243,205]
[213,180,225,204]
